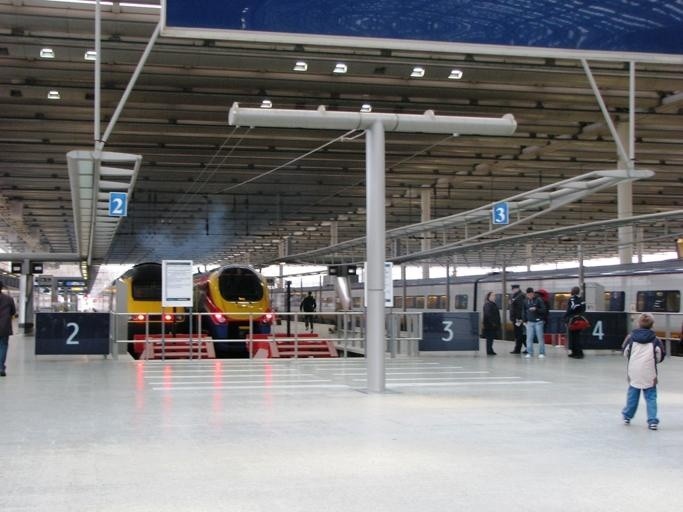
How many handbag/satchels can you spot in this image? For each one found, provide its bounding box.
[569,315,590,331]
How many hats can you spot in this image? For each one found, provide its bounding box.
[512,285,520,287]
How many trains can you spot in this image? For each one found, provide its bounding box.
[93,263,186,352]
[186,265,273,359]
[268,258,683,357]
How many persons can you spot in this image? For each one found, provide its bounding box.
[622,313,666,431]
[300,291,316,333]
[563,286,586,359]
[0,280,16,376]
[483,292,501,355]
[509,284,550,359]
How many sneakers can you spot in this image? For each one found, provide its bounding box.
[567,352,583,358]
[624,417,630,423]
[487,351,496,355]
[306,327,313,334]
[511,350,544,358]
[0,368,6,376]
[649,423,657,430]
